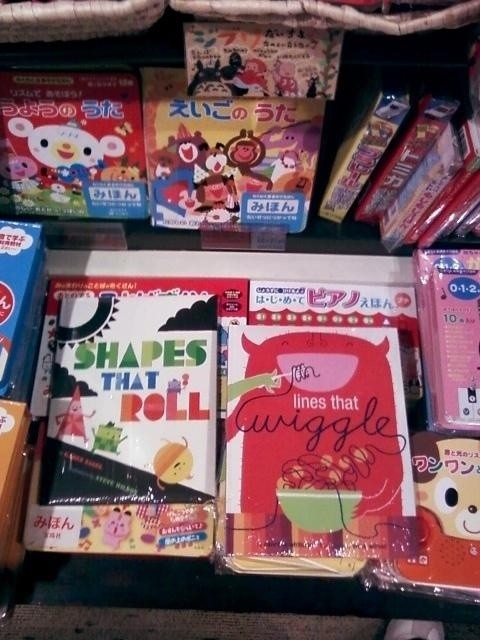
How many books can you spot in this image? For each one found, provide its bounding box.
[0,400,30,570]
[0,58,149,220]
[372,430,480,594]
[318,89,480,254]
[142,64,325,232]
[411,248,480,437]
[0,218,48,399]
